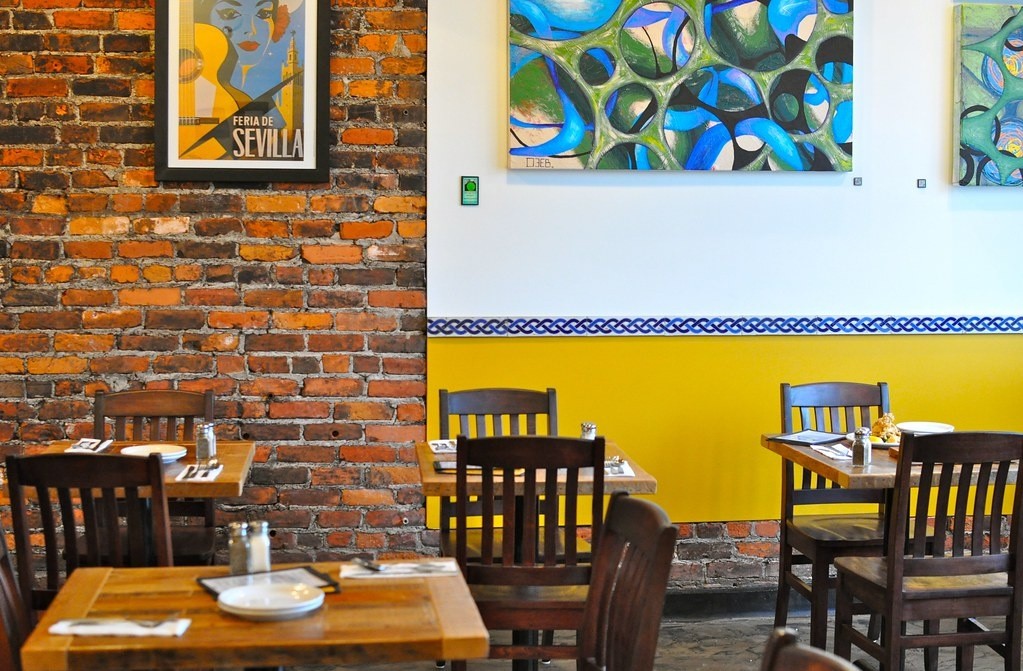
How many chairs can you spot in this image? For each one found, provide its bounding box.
[6,453,174,628]
[438,389,565,560]
[761,626,865,671]
[452,490,679,671]
[0,523,40,671]
[452,432,605,670]
[61,389,219,565]
[774,380,947,651]
[834,432,1023,670]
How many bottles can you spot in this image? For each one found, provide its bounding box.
[206,423,217,457]
[227,521,250,575]
[579,422,597,443]
[857,426,872,464]
[249,521,272,574]
[851,431,866,467]
[195,424,212,460]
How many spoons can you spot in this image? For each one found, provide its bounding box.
[611,455,625,475]
[202,458,220,477]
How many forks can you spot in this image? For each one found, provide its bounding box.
[605,456,617,474]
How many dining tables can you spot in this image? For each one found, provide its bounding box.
[19,558,491,671]
[415,443,658,669]
[760,433,1023,671]
[3,439,256,564]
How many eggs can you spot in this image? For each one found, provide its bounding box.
[888,437,897,443]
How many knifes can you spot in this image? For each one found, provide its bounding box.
[181,466,194,480]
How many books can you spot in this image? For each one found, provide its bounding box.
[433,460,526,476]
[196,566,340,600]
[766,429,846,446]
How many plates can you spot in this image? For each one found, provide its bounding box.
[120,443,187,465]
[897,420,954,435]
[845,431,905,449]
[217,582,326,623]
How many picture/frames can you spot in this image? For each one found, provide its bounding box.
[154,0,330,182]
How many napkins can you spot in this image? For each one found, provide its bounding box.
[175,463,223,482]
[603,460,636,478]
[63,437,114,454]
[811,441,853,460]
[338,564,458,577]
[47,618,191,636]
